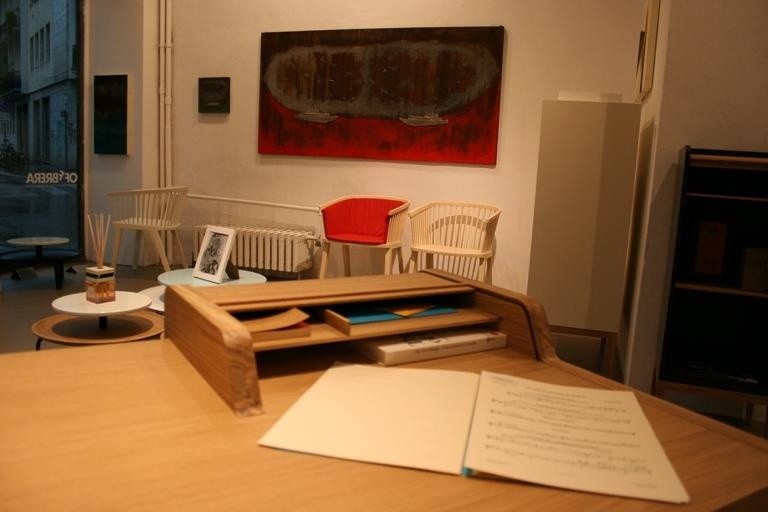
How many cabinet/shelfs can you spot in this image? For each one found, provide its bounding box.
[650,144,768,424]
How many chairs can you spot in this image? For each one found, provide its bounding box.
[318,196,501,283]
[107,186,191,276]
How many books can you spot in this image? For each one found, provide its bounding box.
[348,326,507,366]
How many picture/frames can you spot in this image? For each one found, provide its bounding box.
[193,226,240,282]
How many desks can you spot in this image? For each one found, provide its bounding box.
[0,268,767,512]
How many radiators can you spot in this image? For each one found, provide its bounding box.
[195,225,312,273]
